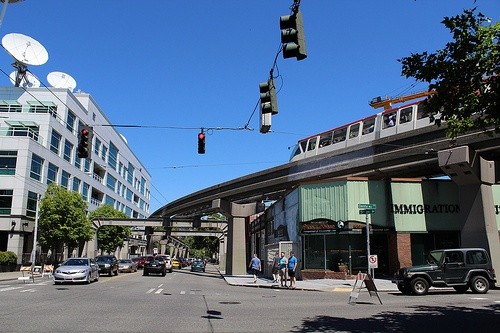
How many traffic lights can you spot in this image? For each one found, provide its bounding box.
[258,78,278,115]
[198,132,205,154]
[280,10,308,60]
[77,126,94,158]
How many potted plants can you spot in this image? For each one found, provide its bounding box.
[339,261,350,275]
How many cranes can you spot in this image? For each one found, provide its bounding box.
[368,77,439,111]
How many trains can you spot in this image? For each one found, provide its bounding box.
[288,83,495,163]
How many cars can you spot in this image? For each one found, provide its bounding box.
[130,255,150,270]
[117,259,137,273]
[54,257,99,285]
[170,257,206,269]
[190,260,206,273]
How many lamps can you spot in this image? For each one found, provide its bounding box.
[12,220,16,226]
[22,222,28,227]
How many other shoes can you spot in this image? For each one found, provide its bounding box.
[253,279,257,283]
[293,286,296,288]
[285,285,287,287]
[273,280,276,283]
[276,281,278,283]
[289,286,292,287]
[281,284,284,287]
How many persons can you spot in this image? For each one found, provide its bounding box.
[272,251,280,283]
[287,251,298,288]
[248,254,261,283]
[278,252,288,288]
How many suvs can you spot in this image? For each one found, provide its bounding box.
[163,255,173,273]
[392,247,497,296]
[94,255,119,277]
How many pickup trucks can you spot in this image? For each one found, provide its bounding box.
[143,256,169,277]
